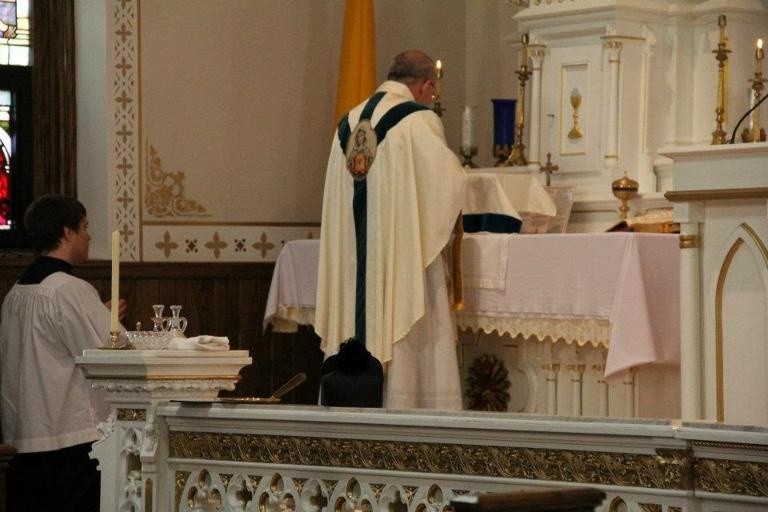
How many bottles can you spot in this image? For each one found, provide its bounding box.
[153,304,187,334]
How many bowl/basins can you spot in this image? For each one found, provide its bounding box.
[126,331,173,349]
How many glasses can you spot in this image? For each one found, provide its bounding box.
[430,82,439,101]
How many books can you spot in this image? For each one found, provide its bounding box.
[604,207,680,233]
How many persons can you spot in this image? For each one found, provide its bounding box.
[0,193,128,512]
[314,50,469,410]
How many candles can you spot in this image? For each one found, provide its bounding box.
[719,15,724,46]
[521,46,528,66]
[755,37,763,74]
[110,228,121,336]
[460,106,472,155]
[434,59,443,102]
